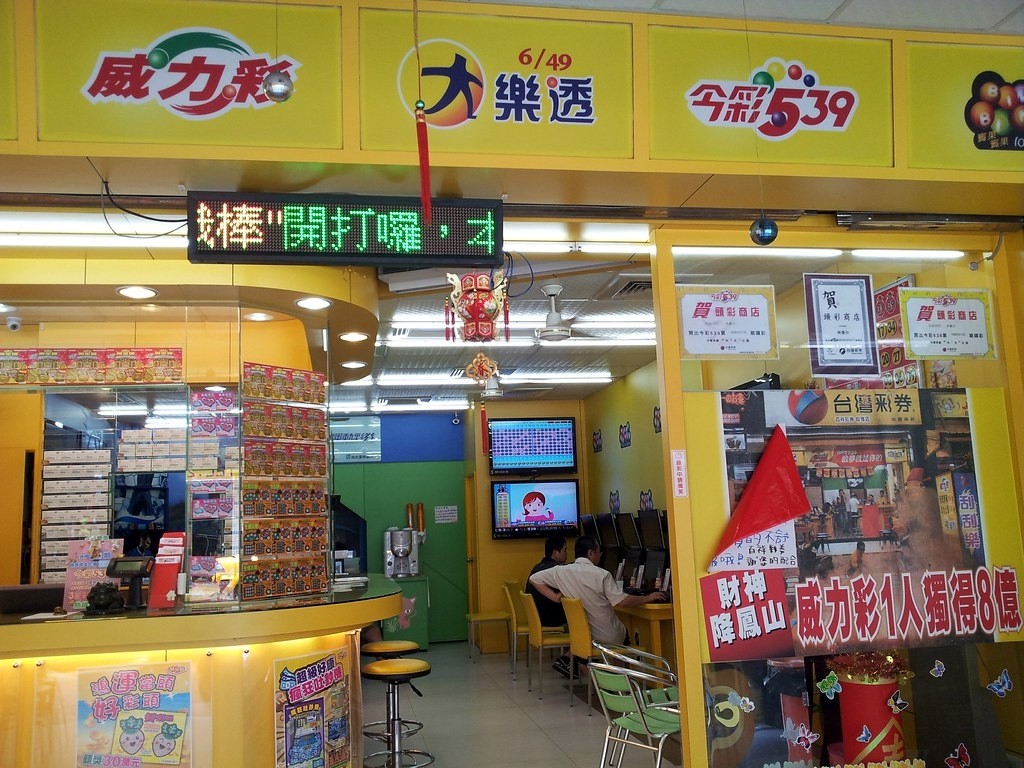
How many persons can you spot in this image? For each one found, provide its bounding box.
[847,541,869,576]
[800,487,888,532]
[523,538,581,679]
[529,535,667,669]
[362,620,387,662]
[126,534,153,557]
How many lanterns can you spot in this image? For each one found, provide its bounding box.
[445,266,509,457]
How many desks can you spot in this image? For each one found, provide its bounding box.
[613,603,674,688]
[857,504,897,514]
[795,515,834,543]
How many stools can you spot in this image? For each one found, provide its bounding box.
[359,641,435,768]
[851,515,860,534]
[817,533,831,553]
[878,529,897,547]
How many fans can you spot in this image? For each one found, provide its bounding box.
[440,374,554,402]
[534,285,596,340]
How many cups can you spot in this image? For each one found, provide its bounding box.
[616,581,623,594]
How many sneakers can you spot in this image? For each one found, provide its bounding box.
[560,655,577,675]
[552,658,579,679]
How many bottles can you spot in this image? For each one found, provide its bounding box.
[654,578,661,592]
[630,576,636,590]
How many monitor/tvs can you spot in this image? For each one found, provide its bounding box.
[729,373,780,390]
[580,509,666,551]
[487,417,577,477]
[490,481,581,540]
[108,472,186,540]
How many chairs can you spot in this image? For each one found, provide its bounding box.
[465,577,648,716]
[587,639,711,768]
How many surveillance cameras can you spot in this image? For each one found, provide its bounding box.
[7,317,21,331]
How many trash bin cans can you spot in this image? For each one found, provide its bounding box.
[767,657,810,729]
